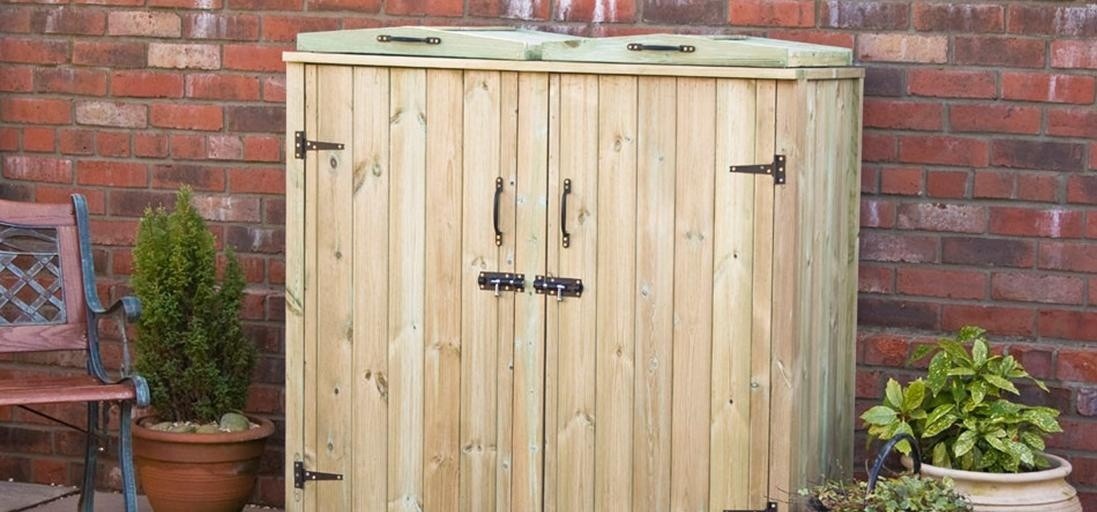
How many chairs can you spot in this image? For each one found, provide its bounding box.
[0,194,149,512]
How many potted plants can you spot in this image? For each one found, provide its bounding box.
[126,186,277,512]
[817,436,977,512]
[863,324,1085,512]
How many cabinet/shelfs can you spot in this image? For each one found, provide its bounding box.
[285,26,867,512]
